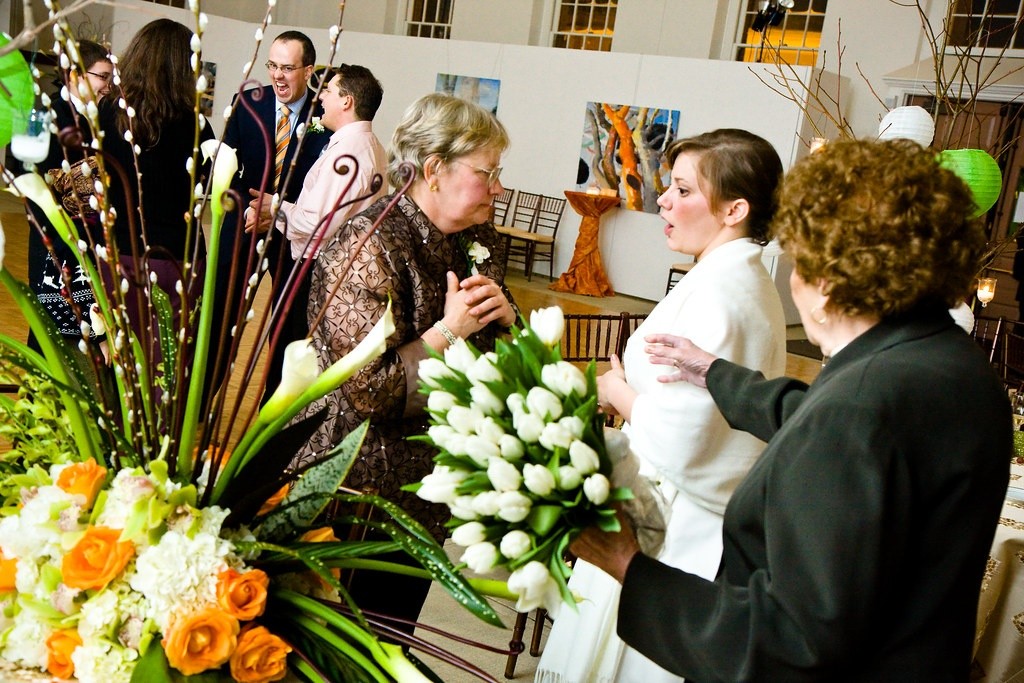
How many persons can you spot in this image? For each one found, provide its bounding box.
[534,126,786,683]
[245,63,389,411]
[185,30,334,422]
[5,39,114,355]
[252,94,526,654]
[569,138,1014,683]
[97,19,215,423]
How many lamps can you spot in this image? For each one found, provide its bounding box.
[751,0,795,32]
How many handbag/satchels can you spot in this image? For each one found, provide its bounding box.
[33,249,114,344]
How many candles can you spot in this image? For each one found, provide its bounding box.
[977,277,997,306]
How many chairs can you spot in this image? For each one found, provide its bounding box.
[504,195,566,283]
[489,188,515,247]
[666,255,698,297]
[553,313,627,429]
[493,191,542,274]
[622,312,650,352]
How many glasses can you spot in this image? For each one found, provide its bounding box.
[448,160,503,187]
[85,70,114,82]
[265,59,305,73]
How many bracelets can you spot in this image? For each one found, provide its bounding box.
[435,319,456,345]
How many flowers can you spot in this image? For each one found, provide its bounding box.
[0,0,671,683]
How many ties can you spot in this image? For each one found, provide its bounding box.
[272,106,292,193]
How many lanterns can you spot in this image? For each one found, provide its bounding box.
[941,148,1003,220]
[880,105,935,149]
